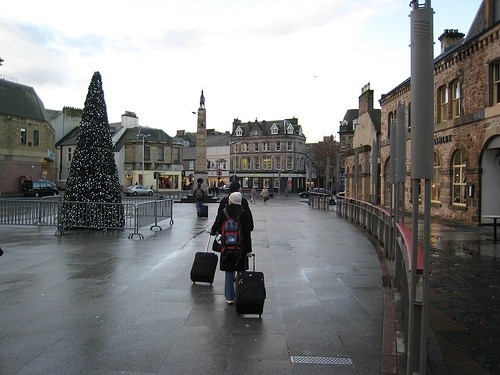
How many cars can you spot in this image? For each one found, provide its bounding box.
[217,184,229,192]
[298,188,333,199]
[124,186,154,197]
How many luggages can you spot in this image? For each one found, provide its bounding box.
[235,252,265,318]
[190,230,219,286]
[199,201,208,217]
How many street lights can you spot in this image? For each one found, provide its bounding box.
[136,133,151,187]
[218,156,221,184]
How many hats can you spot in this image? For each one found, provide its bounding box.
[229,191,243,205]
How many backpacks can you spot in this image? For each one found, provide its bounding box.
[221,206,244,253]
[195,188,203,199]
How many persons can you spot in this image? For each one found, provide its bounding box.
[209,191,254,304]
[217,181,254,281]
[193,183,269,218]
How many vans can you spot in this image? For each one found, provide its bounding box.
[22,179,60,197]
[56,180,68,190]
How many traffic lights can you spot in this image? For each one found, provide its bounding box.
[154,172,162,179]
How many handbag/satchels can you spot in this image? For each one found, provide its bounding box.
[266,196,269,200]
